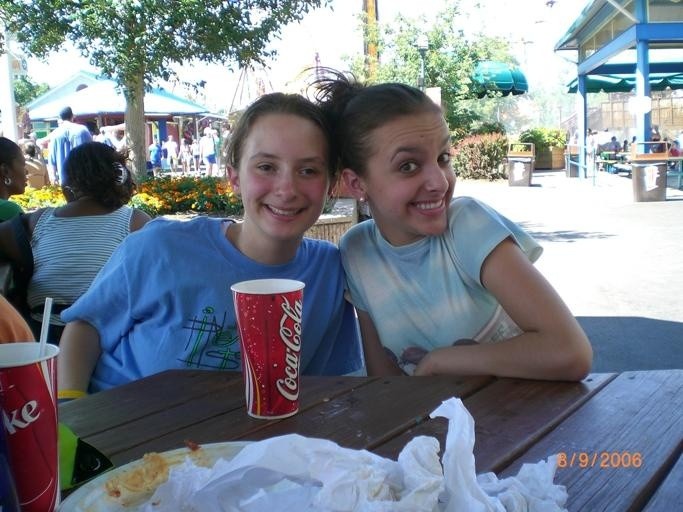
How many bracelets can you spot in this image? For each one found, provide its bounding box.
[56,389,88,399]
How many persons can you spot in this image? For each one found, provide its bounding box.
[53,92,362,402]
[586,126,682,172]
[0,137,32,319]
[298,66,593,383]
[1,141,152,347]
[16,106,232,186]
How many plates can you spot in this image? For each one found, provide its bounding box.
[58,439,117,493]
[54,439,259,511]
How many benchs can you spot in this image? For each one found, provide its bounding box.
[595,151,683,189]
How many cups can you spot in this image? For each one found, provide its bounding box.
[229,277,305,422]
[0,343,62,511]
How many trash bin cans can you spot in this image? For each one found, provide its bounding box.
[629,142,670,202]
[564,144,580,177]
[507,143,536,186]
